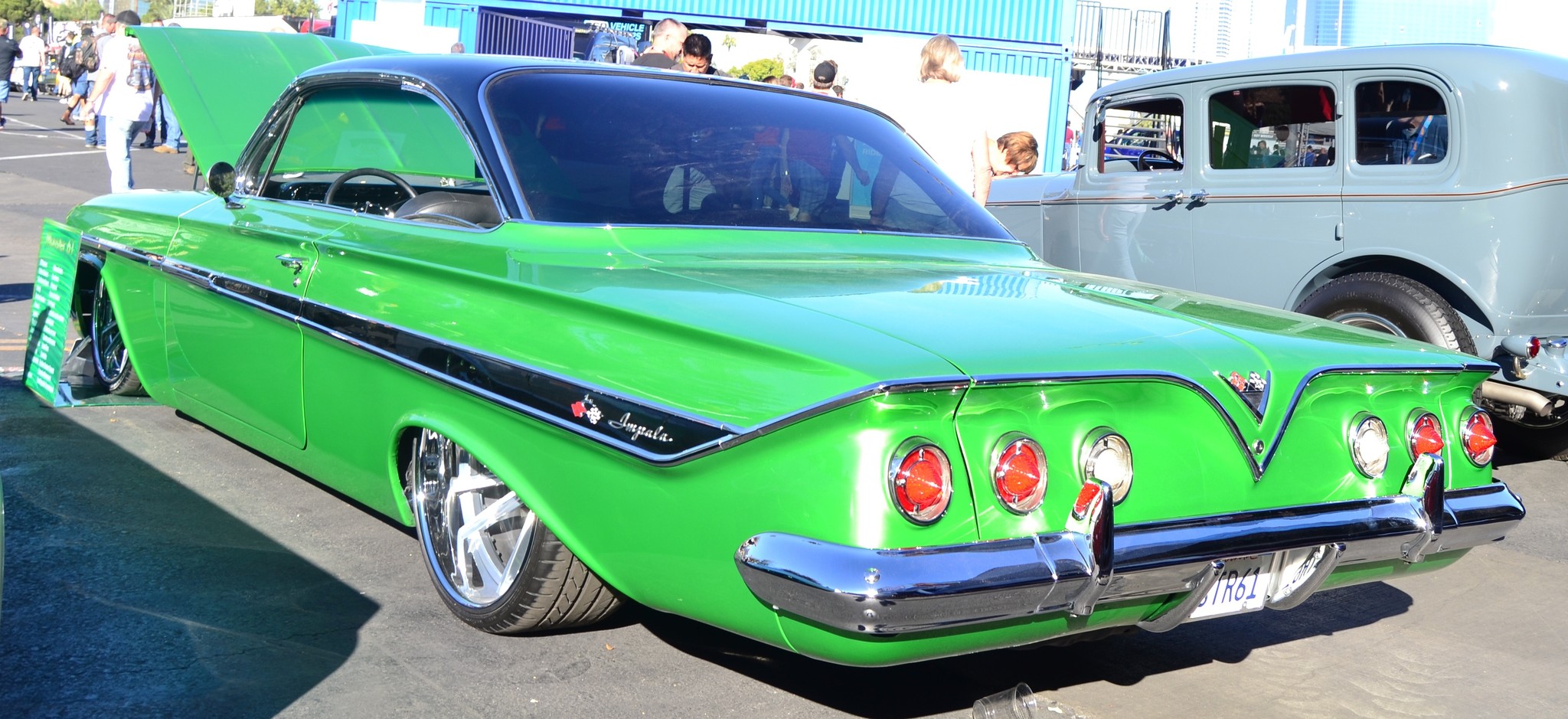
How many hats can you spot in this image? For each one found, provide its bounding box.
[107,9,141,26]
[814,61,836,84]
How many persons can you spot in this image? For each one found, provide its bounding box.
[281,9,316,33]
[80,9,160,194]
[983,130,1040,175]
[1384,104,1449,164]
[450,42,465,54]
[0,17,23,130]
[537,15,871,228]
[1061,120,1141,171]
[39,42,53,68]
[868,35,991,238]
[1248,112,1334,167]
[14,27,46,101]
[1155,125,1178,162]
[53,9,203,176]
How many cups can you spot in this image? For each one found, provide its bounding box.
[84,112,96,131]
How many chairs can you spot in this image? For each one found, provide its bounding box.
[393,191,503,230]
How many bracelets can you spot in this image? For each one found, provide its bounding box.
[870,210,886,218]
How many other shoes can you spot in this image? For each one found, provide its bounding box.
[59,98,67,104]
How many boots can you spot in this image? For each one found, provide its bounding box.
[60,109,75,126]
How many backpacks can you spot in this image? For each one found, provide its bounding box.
[59,42,87,79]
[75,33,112,71]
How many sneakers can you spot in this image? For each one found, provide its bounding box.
[138,139,154,147]
[183,165,202,176]
[30,96,38,101]
[153,144,178,155]
[0,117,6,130]
[21,92,29,101]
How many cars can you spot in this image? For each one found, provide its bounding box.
[65,28,1527,672]
[986,39,1568,438]
